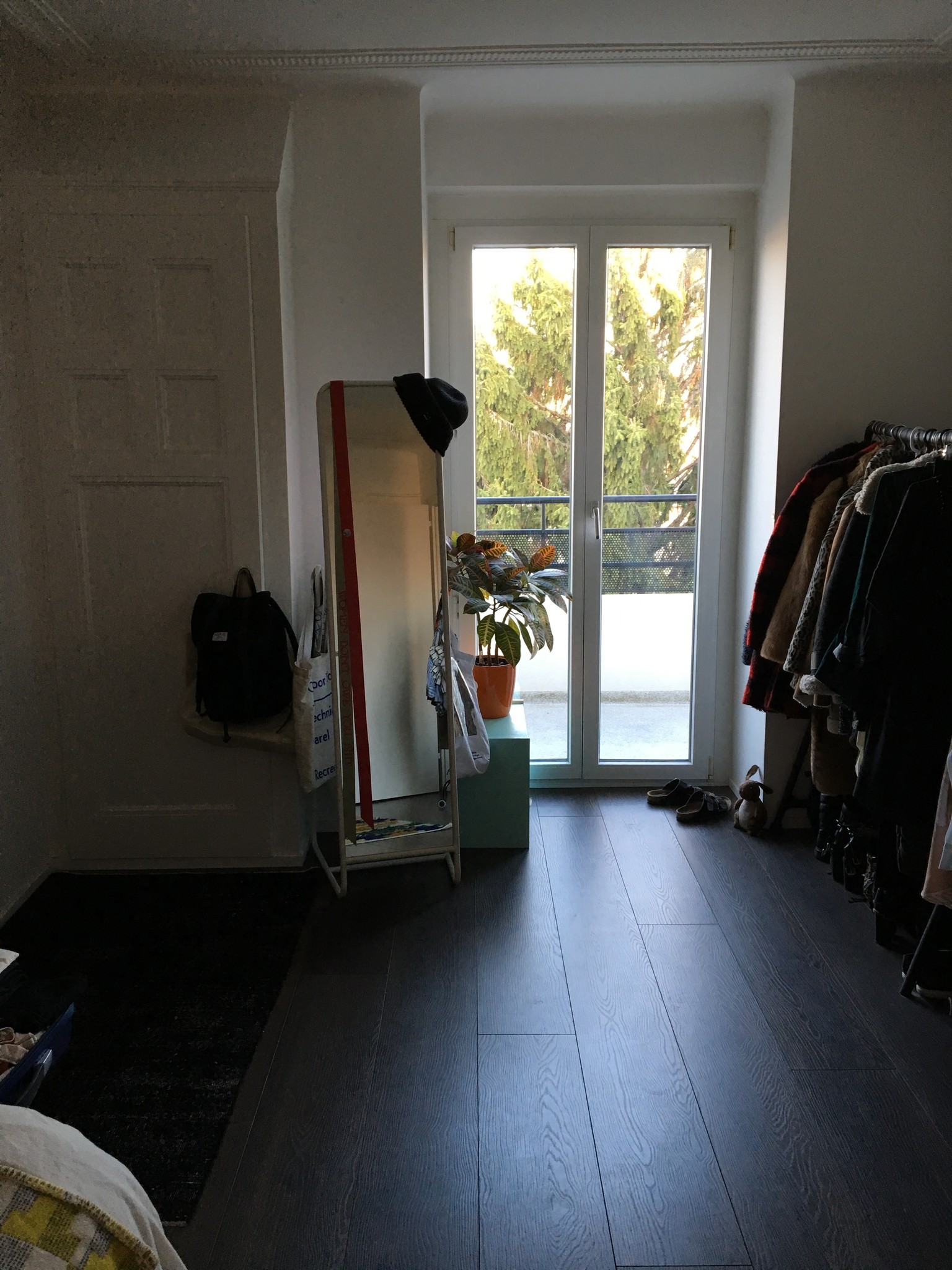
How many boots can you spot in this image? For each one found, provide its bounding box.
[814,793,838,862]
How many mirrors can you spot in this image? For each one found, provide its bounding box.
[316,379,460,862]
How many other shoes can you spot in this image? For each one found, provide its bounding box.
[830,823,864,894]
[901,949,952,998]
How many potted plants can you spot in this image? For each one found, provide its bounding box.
[445,531,574,719]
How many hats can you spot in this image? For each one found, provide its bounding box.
[394,372,468,457]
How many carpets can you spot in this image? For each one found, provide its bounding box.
[345,817,452,845]
[0,873,318,1226]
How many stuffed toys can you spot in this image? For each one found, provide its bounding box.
[733,781,774,837]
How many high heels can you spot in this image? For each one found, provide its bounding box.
[872,888,928,945]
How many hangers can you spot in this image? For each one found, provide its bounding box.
[871,421,952,458]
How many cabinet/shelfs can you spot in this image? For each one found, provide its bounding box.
[460,699,531,849]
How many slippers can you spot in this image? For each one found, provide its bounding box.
[676,789,732,822]
[646,777,703,804]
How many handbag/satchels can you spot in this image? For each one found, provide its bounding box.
[295,564,337,793]
[190,569,299,726]
[439,612,490,778]
